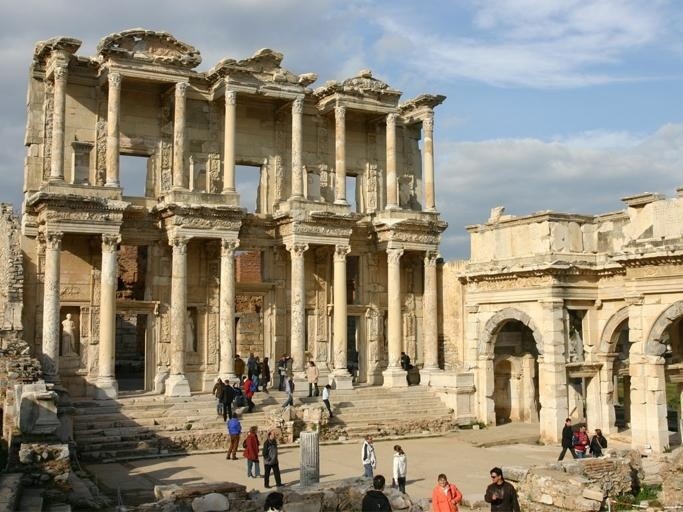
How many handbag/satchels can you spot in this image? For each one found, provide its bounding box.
[243,440,246,448]
[249,384,256,392]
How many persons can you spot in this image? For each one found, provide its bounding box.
[361,434,377,477]
[391,444,407,493]
[361,475,392,511]
[571,425,590,458]
[212,352,295,421]
[431,473,462,511]
[483,467,520,511]
[589,429,608,458]
[263,491,284,511]
[401,351,411,385]
[225,412,240,460]
[242,425,261,479]
[60,312,75,357]
[261,430,285,489]
[558,418,578,460]
[321,384,333,418]
[305,361,320,397]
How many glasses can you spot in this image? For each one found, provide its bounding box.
[492,476,496,479]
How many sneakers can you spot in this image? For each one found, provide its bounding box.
[226,458,238,460]
[265,483,284,488]
[248,475,264,478]
